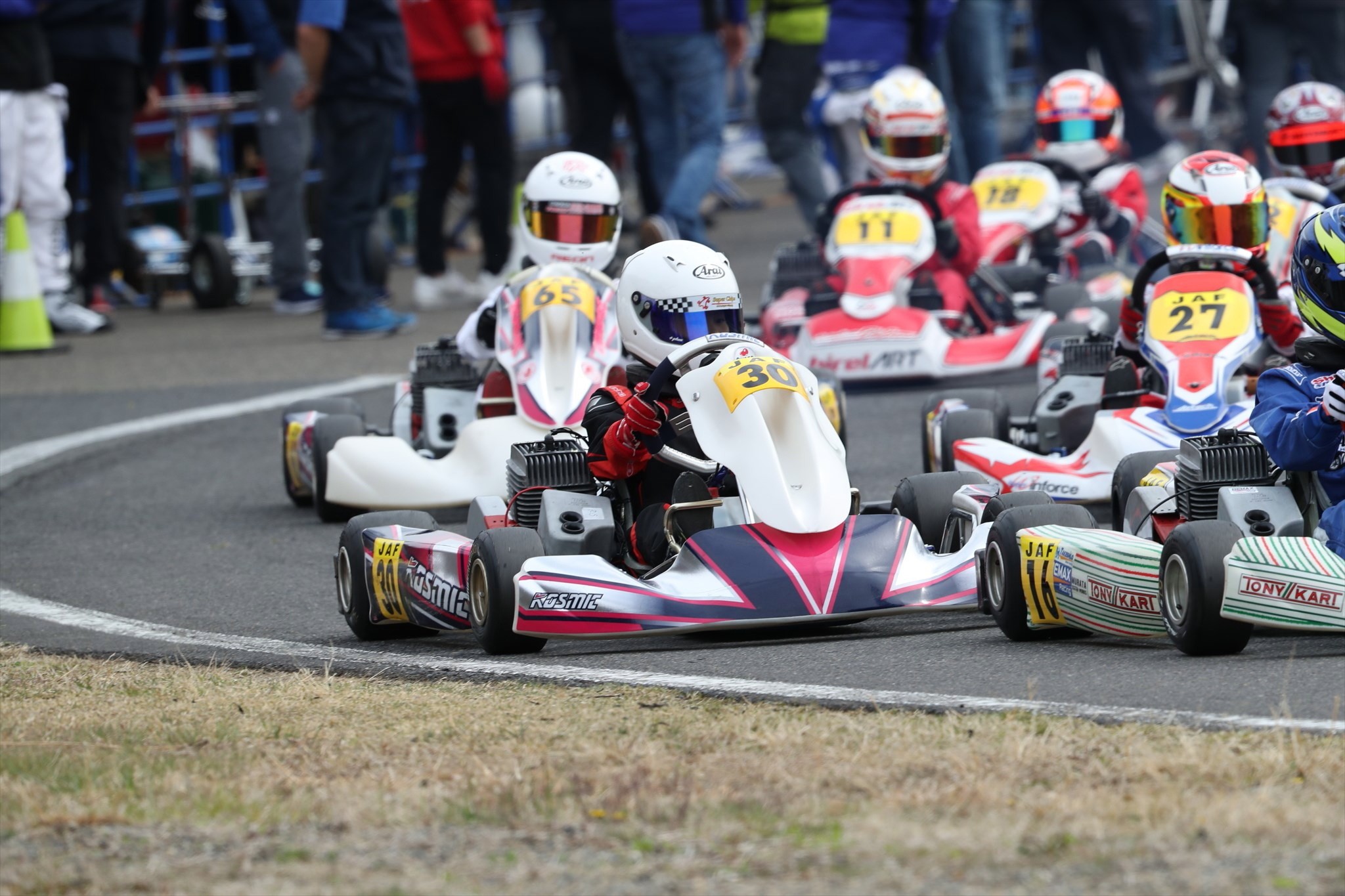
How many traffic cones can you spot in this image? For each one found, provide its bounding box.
[0,209,72,356]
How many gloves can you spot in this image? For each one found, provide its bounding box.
[933,218,957,257]
[475,284,514,347]
[1258,298,1304,349]
[1320,368,1345,423]
[1079,184,1109,224]
[1118,295,1143,344]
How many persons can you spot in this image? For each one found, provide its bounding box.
[763,67,1345,409]
[0,0,1345,336]
[580,240,749,579]
[1249,202,1345,559]
[455,151,625,418]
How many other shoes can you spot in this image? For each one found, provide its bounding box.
[51,300,111,336]
[477,260,513,297]
[415,268,474,301]
[271,282,328,315]
[640,213,673,241]
[325,303,409,332]
[1100,358,1142,409]
[670,468,717,542]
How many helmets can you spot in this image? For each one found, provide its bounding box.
[521,149,623,271]
[857,66,951,188]
[1032,67,1123,169]
[1265,81,1345,184]
[1160,150,1271,264]
[617,239,744,380]
